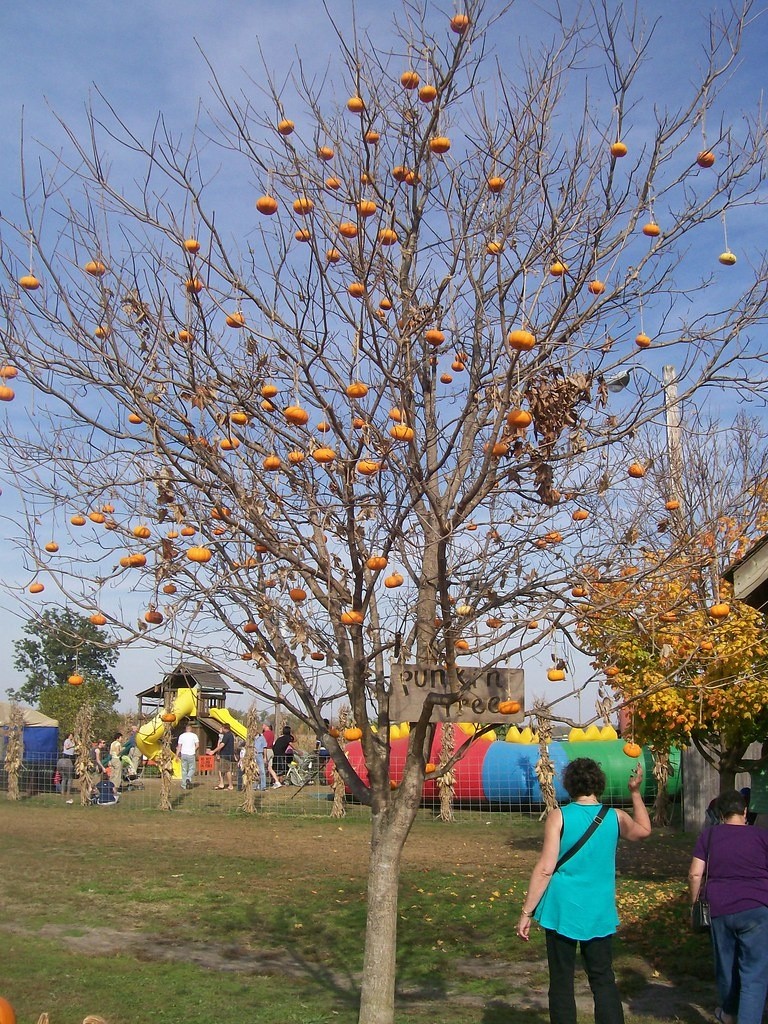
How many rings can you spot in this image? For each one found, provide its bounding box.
[516,933,520,938]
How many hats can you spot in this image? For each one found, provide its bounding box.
[740,788,751,800]
[263,721,270,726]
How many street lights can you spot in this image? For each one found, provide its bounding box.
[606,365,678,536]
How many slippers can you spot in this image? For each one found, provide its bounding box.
[715,1007,736,1024]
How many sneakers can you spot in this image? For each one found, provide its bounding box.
[271,782,282,788]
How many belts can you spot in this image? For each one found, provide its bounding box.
[255,751,264,754]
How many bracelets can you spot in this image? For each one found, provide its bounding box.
[521,906,532,917]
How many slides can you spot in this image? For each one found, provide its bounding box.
[135,687,198,780]
[209,707,298,767]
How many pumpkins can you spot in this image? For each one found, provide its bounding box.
[0,996,17,1024]
[1,12,729,790]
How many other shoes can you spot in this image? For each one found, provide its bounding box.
[212,784,224,790]
[261,787,267,790]
[180,785,186,790]
[253,786,256,789]
[186,779,191,786]
[224,786,233,791]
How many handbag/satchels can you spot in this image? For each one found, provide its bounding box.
[285,745,293,755]
[692,899,712,933]
[231,751,239,762]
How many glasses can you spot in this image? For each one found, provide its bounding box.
[101,742,105,744]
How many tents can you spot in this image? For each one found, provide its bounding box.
[0,701,60,792]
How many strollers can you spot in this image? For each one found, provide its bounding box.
[119,755,145,792]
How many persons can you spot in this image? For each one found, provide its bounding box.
[208,718,331,791]
[688,739,768,1024]
[516,758,651,1024]
[54,724,200,806]
